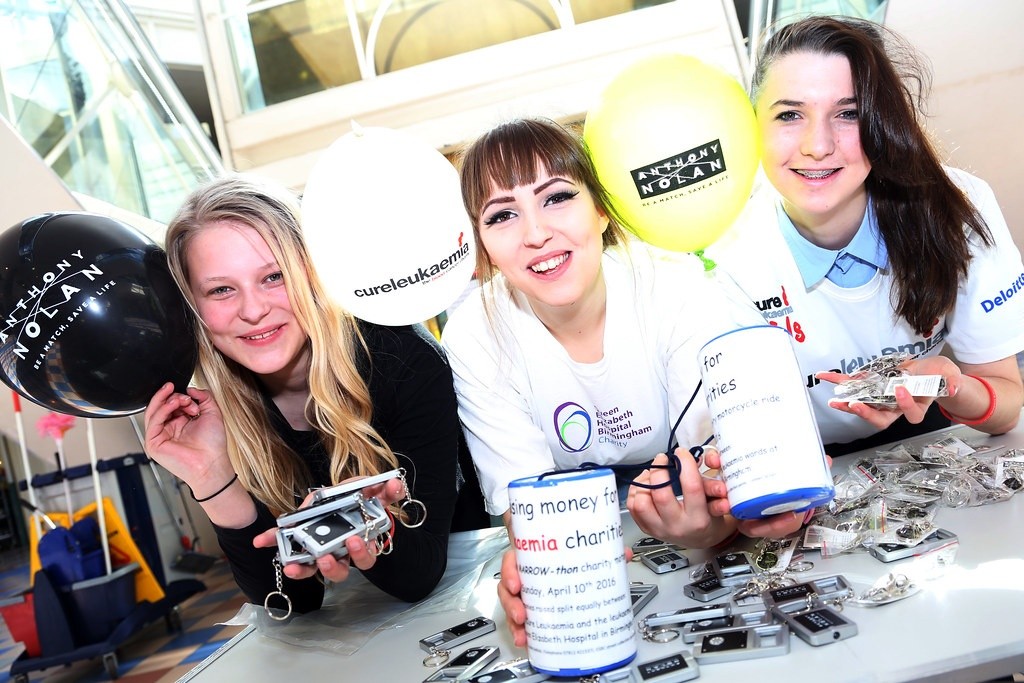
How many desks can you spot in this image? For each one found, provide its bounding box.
[167,410,1024,683]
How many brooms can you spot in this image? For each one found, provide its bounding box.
[128,414,220,574]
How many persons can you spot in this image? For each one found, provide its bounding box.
[441,116,746,647]
[705,14,1024,537]
[145,176,491,616]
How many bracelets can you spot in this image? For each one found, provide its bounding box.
[937,372,996,426]
[191,473,239,502]
